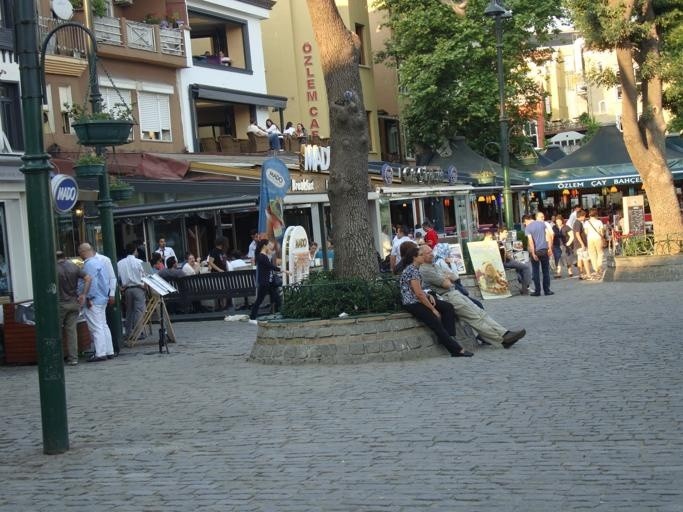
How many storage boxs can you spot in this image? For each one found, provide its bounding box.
[162,269,256,314]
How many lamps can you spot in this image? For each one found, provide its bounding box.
[247,131,271,153]
[283,131,299,152]
[217,135,242,153]
[238,138,250,153]
[196,136,218,152]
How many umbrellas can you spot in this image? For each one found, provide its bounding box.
[452,349,475,357]
[437,335,457,344]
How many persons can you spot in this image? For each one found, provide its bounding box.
[419,244,526,349]
[399,248,473,357]
[483,204,628,296]
[55,229,335,365]
[200,51,213,57]
[378,221,439,275]
[217,50,231,66]
[246,118,307,152]
[433,242,492,345]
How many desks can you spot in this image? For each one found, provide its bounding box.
[481,1,516,232]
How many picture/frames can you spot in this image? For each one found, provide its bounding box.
[297,135,307,139]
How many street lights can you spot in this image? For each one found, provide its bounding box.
[602,237,608,249]
[273,272,283,287]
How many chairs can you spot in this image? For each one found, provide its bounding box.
[67,357,79,365]
[522,287,554,296]
[501,329,526,348]
[86,349,119,362]
[248,318,257,324]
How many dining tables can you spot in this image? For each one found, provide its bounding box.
[519,152,536,165]
[109,178,135,201]
[67,148,109,176]
[61,98,140,145]
[479,168,494,185]
